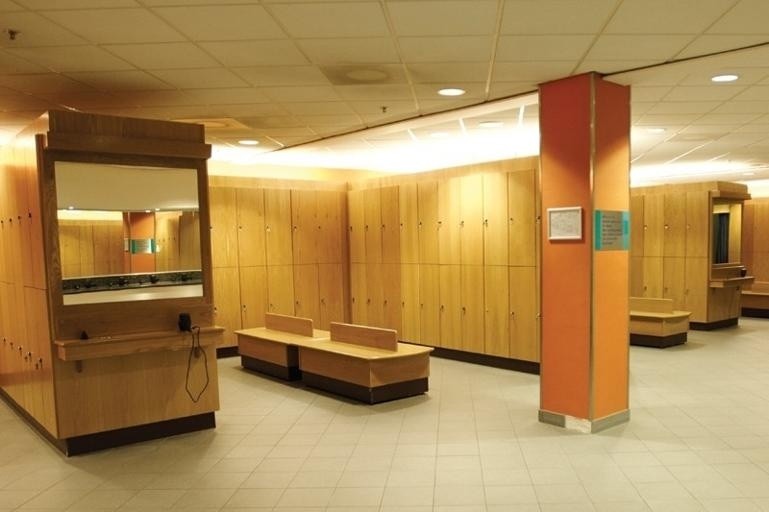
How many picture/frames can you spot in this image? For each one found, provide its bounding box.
[547,206,583,241]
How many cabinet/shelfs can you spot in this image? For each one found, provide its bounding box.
[347,168,541,376]
[629,180,751,331]
[209,186,346,347]
[0,110,225,457]
[742,196,769,291]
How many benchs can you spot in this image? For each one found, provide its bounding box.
[739,281,769,319]
[628,297,692,348]
[232,312,435,406]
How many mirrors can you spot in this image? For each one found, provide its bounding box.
[52,160,207,306]
[712,203,743,264]
